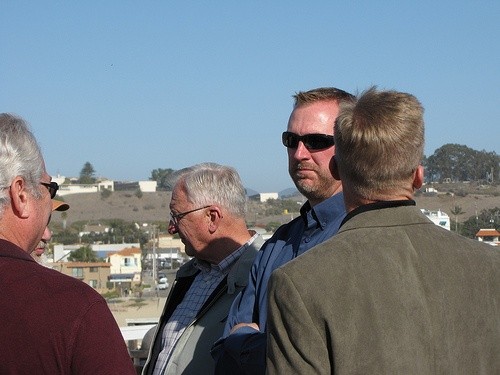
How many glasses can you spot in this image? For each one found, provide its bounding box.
[169,206,211,224]
[283,132,335,150]
[41,182,59,199]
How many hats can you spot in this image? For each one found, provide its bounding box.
[52,199,70,211]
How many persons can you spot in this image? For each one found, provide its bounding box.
[264,86,500,375]
[30,199,70,267]
[210,87,363,374]
[0,111,140,375]
[141,162,266,374]
